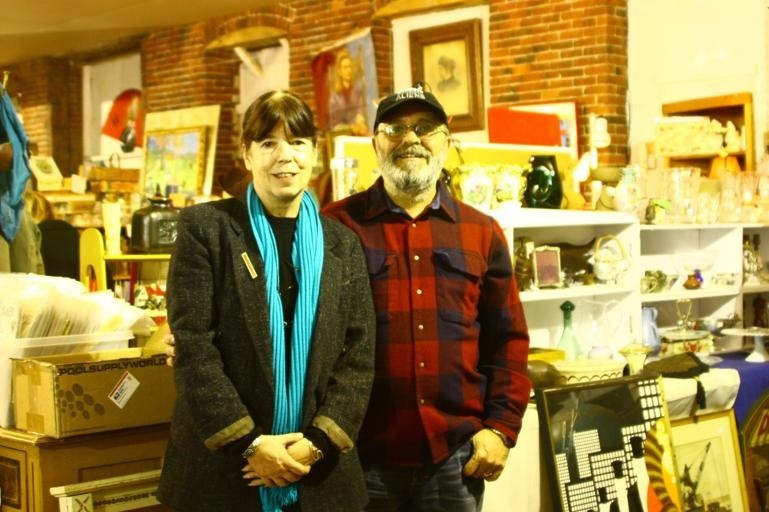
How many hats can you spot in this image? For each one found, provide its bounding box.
[372,89,447,132]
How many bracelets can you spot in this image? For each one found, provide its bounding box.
[304,436,325,461]
[242,434,263,459]
[491,424,512,447]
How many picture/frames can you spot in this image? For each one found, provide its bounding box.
[140,126,206,193]
[409,18,484,132]
[535,371,750,512]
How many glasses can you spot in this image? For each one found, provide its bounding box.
[375,121,451,137]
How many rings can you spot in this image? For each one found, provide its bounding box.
[484,469,494,478]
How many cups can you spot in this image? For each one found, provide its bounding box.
[579,164,768,224]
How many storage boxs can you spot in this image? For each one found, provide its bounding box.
[0,329,175,439]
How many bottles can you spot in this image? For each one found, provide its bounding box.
[555,300,582,359]
[642,306,662,355]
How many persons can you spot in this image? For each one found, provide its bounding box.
[436,56,458,94]
[329,53,369,139]
[156,90,377,512]
[320,90,532,511]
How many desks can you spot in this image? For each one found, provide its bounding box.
[103,254,171,304]
[0,422,170,512]
